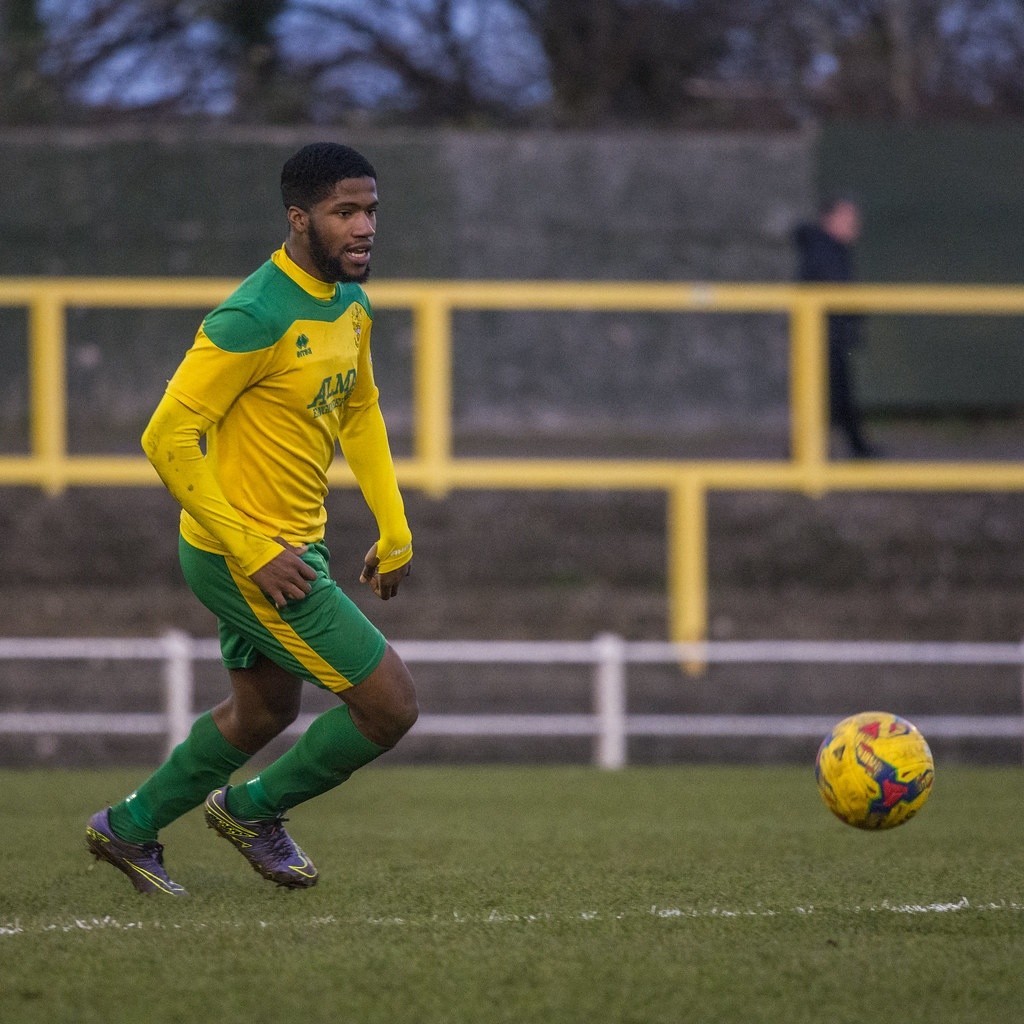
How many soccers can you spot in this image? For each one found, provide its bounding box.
[815,712,938,829]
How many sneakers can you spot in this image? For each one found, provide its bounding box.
[204,784,319,890]
[85,807,191,898]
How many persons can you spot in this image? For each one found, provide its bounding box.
[81,138,419,902]
[790,194,886,462]
